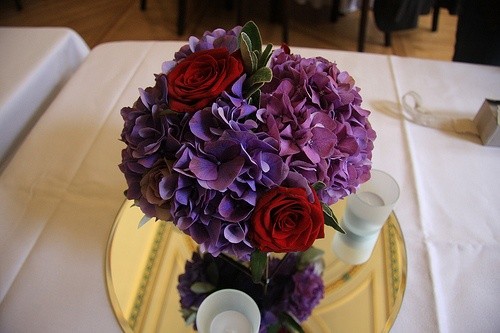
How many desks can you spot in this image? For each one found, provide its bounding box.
[1,26,499,333]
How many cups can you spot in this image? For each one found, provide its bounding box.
[332,169,400,265]
[196,289,262,333]
